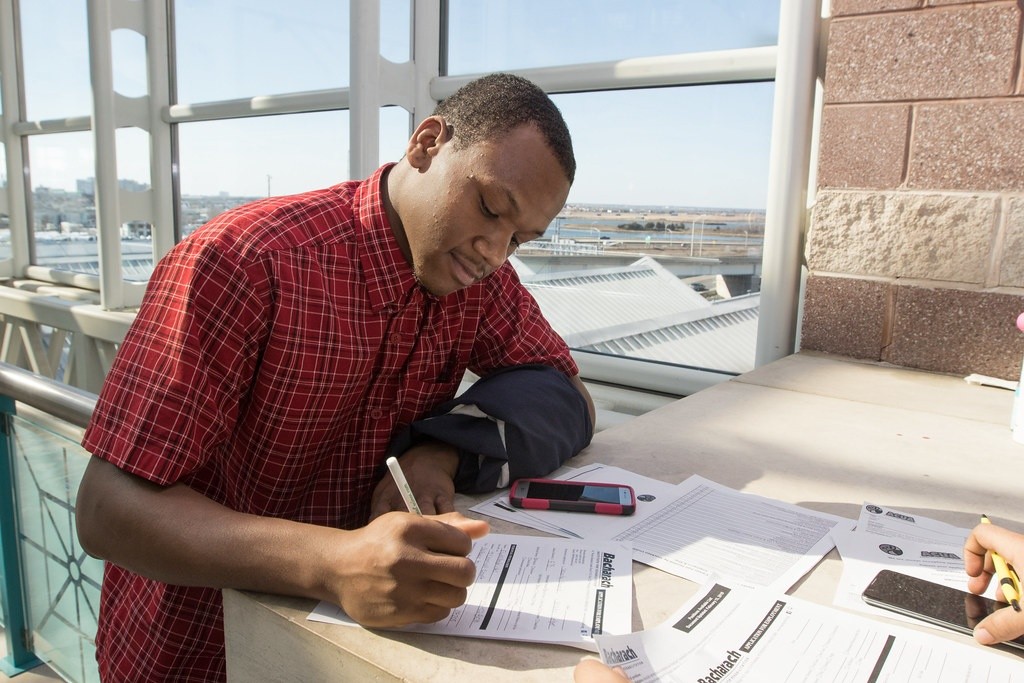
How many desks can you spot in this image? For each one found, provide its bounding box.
[220,353,1024,683]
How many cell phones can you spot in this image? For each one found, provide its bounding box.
[510,478,636,516]
[861,569,1024,650]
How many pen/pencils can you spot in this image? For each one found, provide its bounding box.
[386,456,423,517]
[981,513,1023,612]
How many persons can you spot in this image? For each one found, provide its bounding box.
[74,72,596,683]
[573,521,1024,683]
